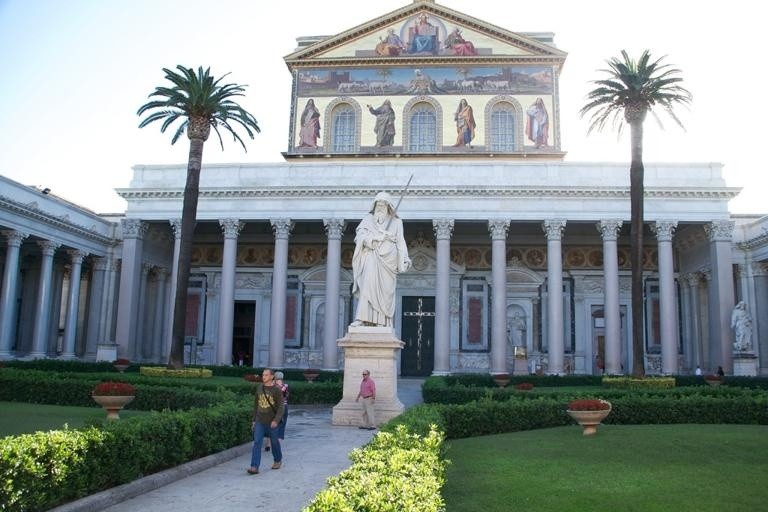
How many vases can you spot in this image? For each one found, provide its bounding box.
[303,374,319,381]
[493,380,510,389]
[706,379,724,387]
[112,363,128,373]
[92,393,136,420]
[566,408,610,433]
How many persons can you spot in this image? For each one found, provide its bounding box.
[695,366,702,385]
[265,371,289,452]
[509,312,526,347]
[452,98,476,148]
[376,29,405,57]
[445,28,477,56]
[410,14,435,53]
[355,369,376,431]
[526,98,549,146]
[717,366,724,376]
[367,99,395,146]
[730,300,753,350]
[350,192,412,327]
[596,355,603,375]
[298,98,320,148]
[247,367,285,473]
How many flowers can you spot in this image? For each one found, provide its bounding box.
[706,374,720,379]
[570,398,606,411]
[305,368,315,373]
[92,382,135,396]
[113,358,129,364]
[494,373,509,381]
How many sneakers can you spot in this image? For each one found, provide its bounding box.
[264,446,271,452]
[247,468,257,474]
[272,461,281,468]
[359,426,375,430]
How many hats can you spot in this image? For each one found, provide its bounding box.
[275,372,283,379]
